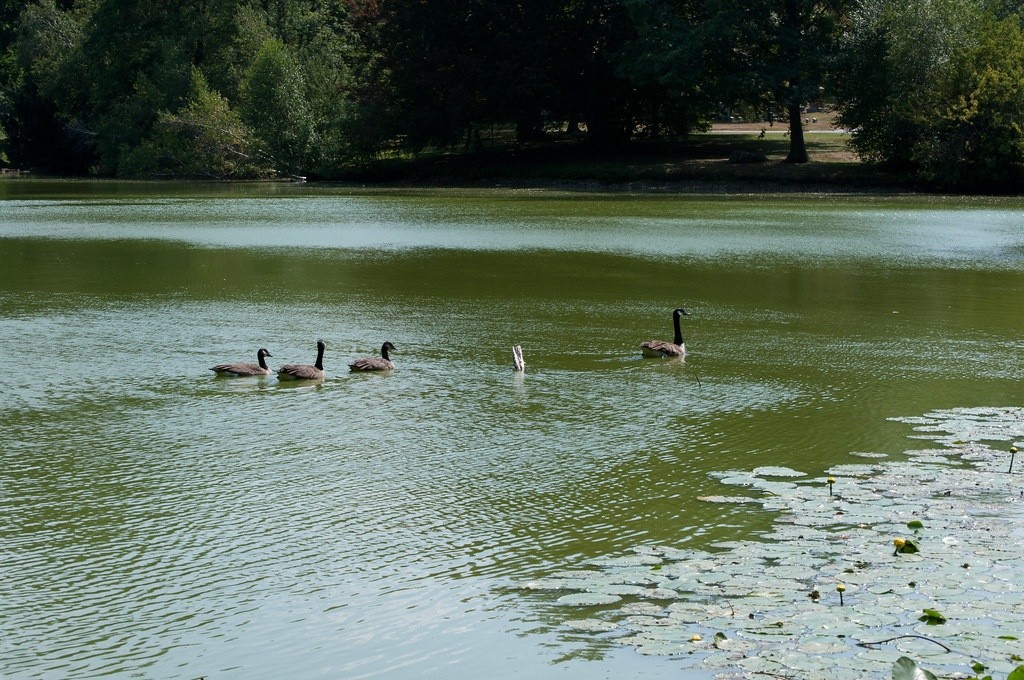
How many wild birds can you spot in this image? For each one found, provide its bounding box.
[276,339,327,379]
[208,348,273,378]
[638,308,692,358]
[347,341,398,371]
